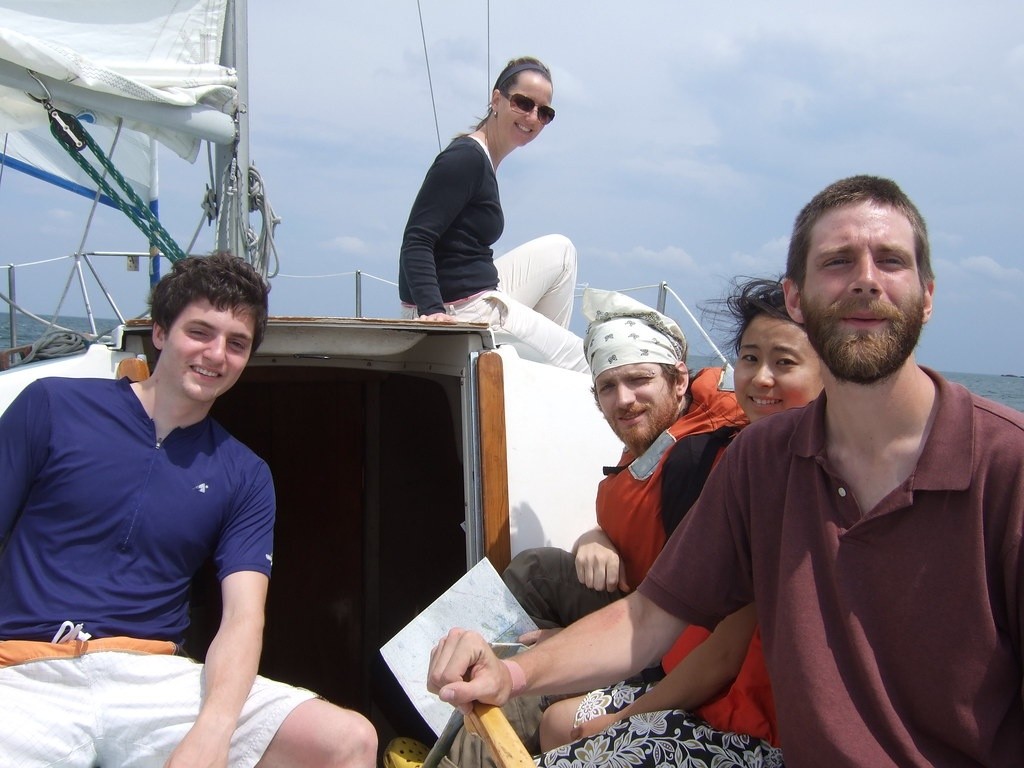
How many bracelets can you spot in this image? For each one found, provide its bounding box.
[501,659,526,699]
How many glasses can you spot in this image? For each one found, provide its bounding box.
[501,91,555,126]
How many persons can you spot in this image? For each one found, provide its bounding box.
[0,248,379,767]
[398,55,591,375]
[426,175,1023,768]
[538,272,824,768]
[420,287,750,768]
